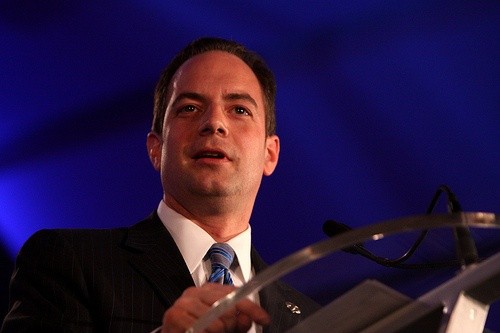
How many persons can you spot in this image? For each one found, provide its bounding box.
[4,35,326,333]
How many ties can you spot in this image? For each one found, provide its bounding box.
[207,243,236,285]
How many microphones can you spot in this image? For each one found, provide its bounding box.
[323,184,479,273]
